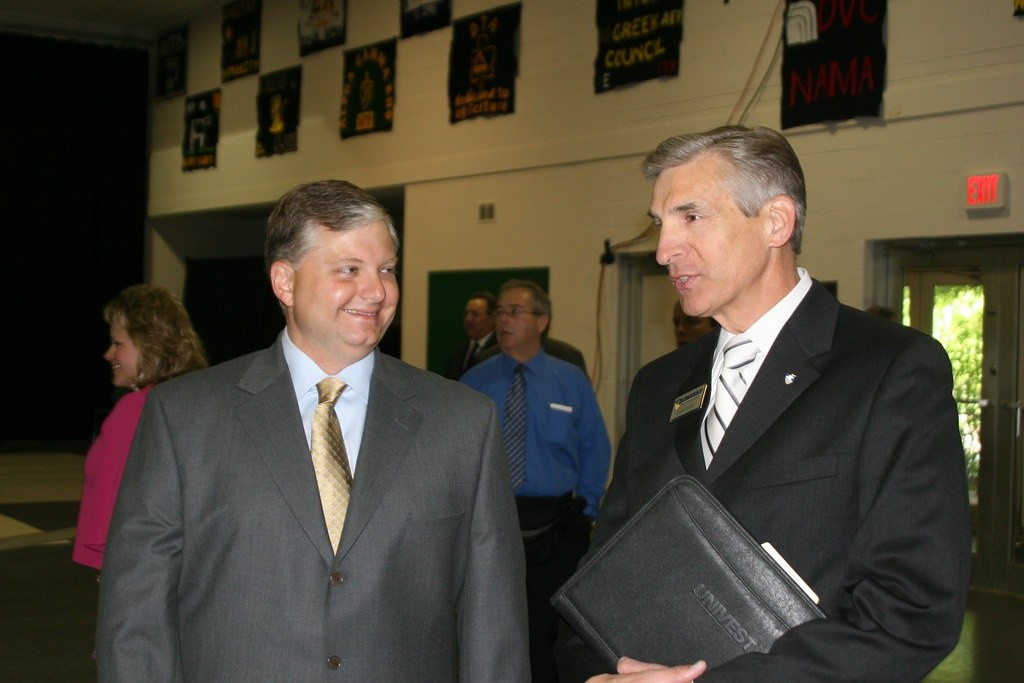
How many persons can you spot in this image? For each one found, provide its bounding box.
[71,286,209,683]
[552,124,972,683]
[444,280,612,683]
[94,179,532,683]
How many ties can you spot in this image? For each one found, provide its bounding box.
[502,364,524,491]
[702,333,758,467]
[310,376,352,558]
[466,342,481,369]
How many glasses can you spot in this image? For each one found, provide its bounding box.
[493,305,545,318]
